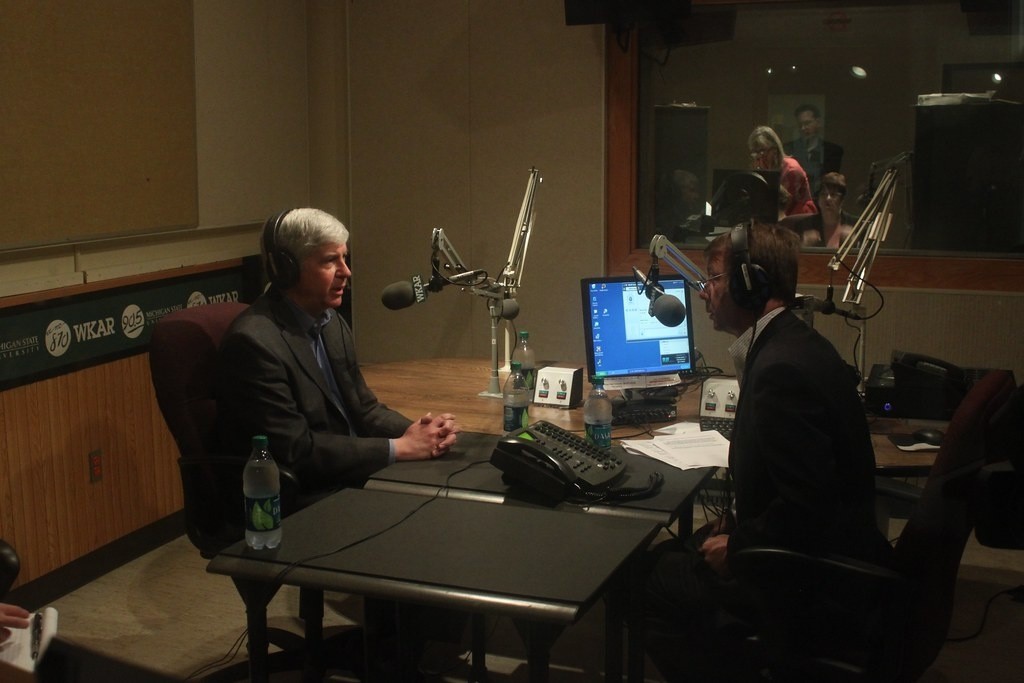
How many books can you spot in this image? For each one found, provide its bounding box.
[0,607,58,683]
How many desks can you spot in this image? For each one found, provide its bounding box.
[204,431,722,683]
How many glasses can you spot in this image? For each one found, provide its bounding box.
[696,270,731,292]
[747,146,773,159]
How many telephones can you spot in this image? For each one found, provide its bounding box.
[490,419,627,497]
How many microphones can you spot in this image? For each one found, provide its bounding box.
[346,280,351,291]
[502,290,519,320]
[855,162,877,211]
[381,269,484,311]
[631,265,686,327]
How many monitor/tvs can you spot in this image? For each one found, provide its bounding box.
[712,169,780,227]
[580,275,697,383]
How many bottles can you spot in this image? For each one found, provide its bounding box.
[503,362,530,436]
[511,331,535,403]
[243,437,281,550]
[582,377,611,454]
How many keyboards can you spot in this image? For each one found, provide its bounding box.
[611,406,677,426]
[700,417,734,441]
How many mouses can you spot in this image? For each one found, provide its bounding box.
[913,429,945,446]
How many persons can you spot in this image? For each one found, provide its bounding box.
[655,171,700,242]
[618,222,894,683]
[216,207,459,683]
[748,103,872,249]
[0,602,30,647]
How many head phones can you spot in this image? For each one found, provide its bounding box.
[264,207,300,291]
[730,222,770,303]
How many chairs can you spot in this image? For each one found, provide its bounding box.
[684,370,1016,683]
[150,303,423,683]
